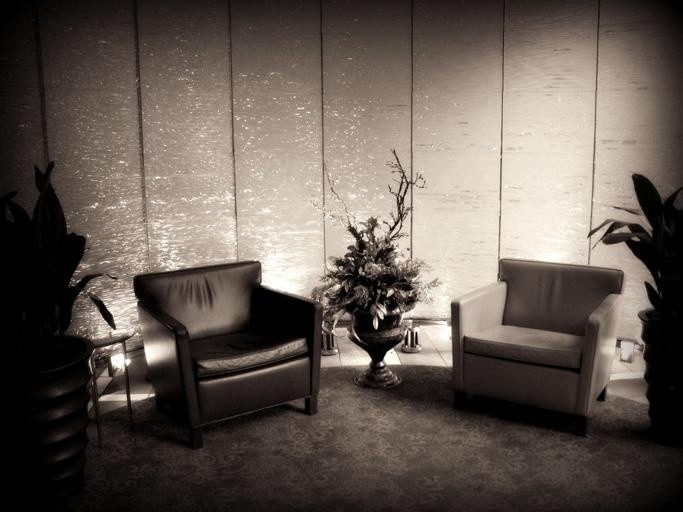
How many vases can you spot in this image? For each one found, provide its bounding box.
[348,309,406,388]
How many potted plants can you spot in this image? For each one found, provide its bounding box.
[0,160,118,511]
[587,171,683,432]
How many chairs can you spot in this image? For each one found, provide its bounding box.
[132,260,322,449]
[451,259,626,437]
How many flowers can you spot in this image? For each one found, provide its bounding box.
[308,148,442,330]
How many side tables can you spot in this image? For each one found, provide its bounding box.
[92,330,134,435]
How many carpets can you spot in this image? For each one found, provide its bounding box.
[100,366,649,512]
